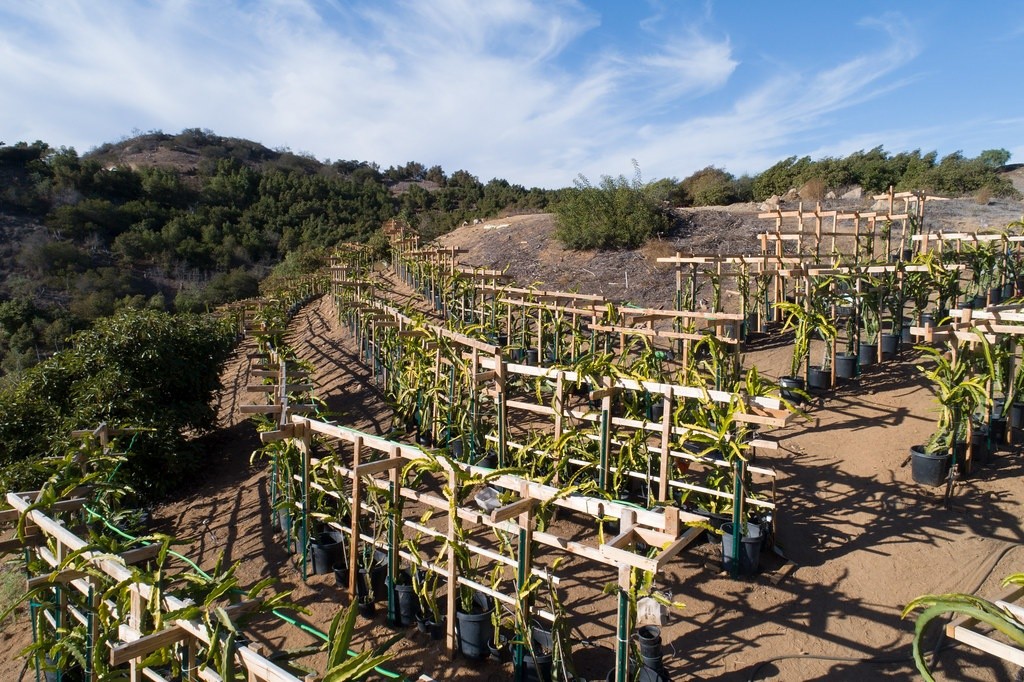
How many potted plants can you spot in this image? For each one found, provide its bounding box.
[0,215,1024,682]
